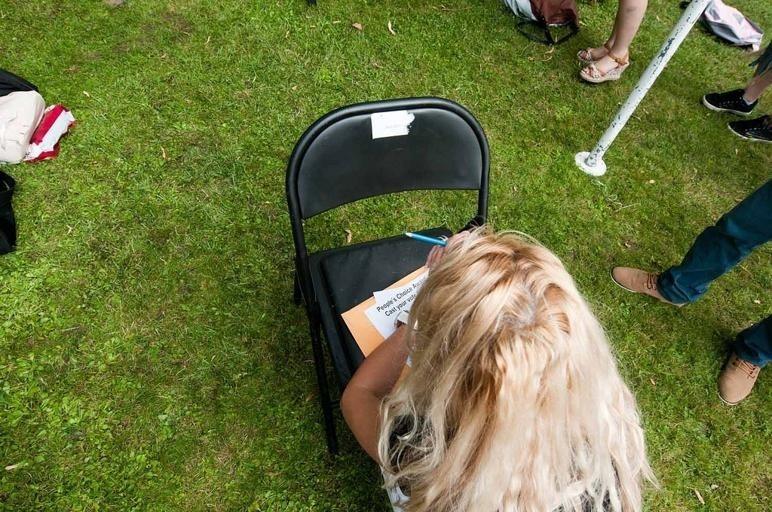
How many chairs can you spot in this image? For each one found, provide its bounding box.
[285,95,491,461]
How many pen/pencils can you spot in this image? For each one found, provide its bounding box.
[404,232,447,247]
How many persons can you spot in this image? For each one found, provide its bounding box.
[339,222,659,512]
[609,178,772,405]
[576,0,649,84]
[702,40,772,143]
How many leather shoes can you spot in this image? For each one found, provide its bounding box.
[609,265,685,309]
[717,339,760,405]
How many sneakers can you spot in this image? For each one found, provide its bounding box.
[702,86,760,117]
[728,114,772,142]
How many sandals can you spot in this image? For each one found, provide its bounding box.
[579,51,630,83]
[577,42,613,63]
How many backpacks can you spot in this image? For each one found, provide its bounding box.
[504,0,578,27]
